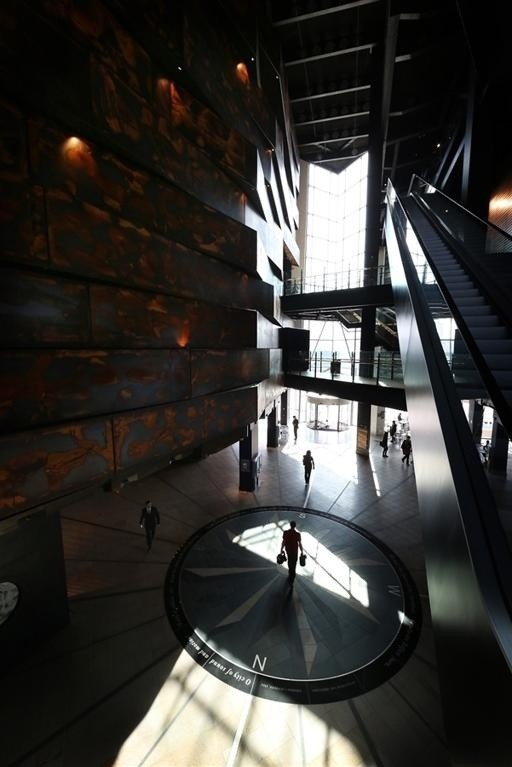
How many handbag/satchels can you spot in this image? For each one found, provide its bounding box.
[300,554,306,567]
[277,552,287,564]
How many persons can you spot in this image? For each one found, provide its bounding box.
[390,421,397,441]
[281,521,307,582]
[292,414,300,440]
[398,413,402,421]
[138,500,161,553]
[400,435,412,466]
[303,451,316,486]
[381,431,389,457]
